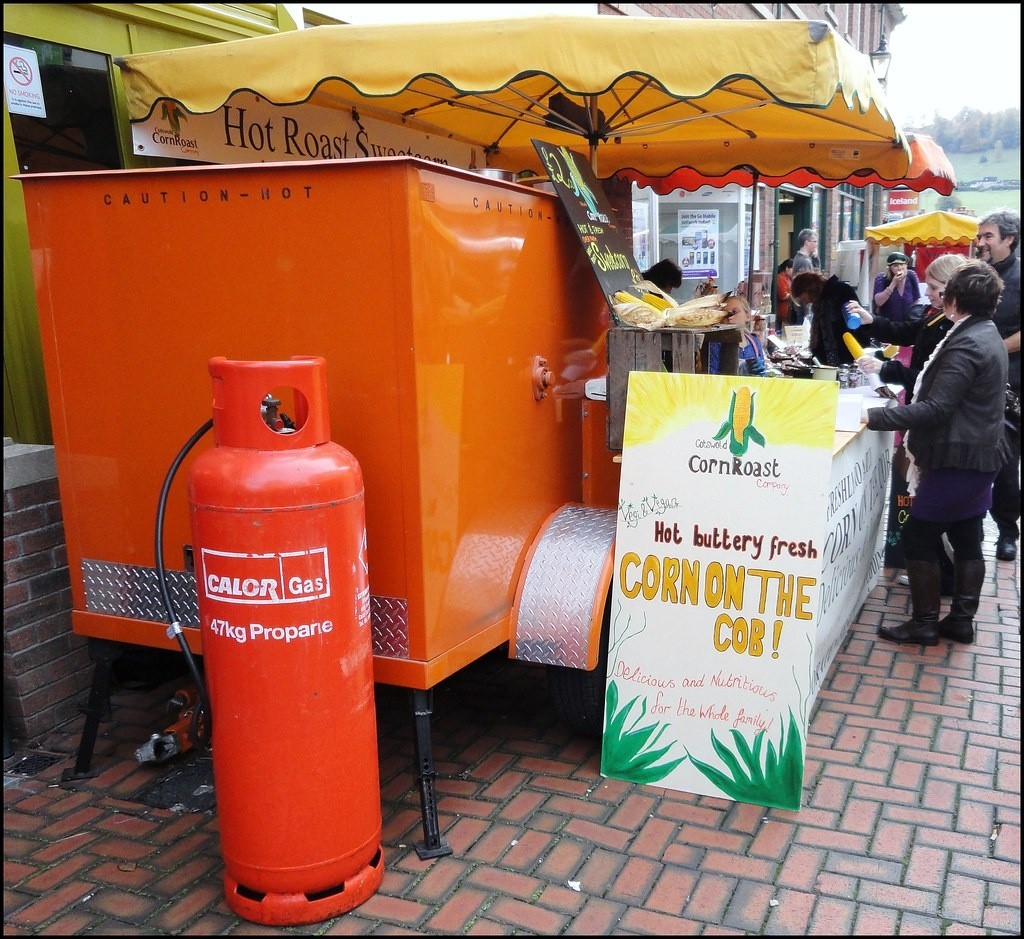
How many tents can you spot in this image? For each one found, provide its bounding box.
[864,211,982,259]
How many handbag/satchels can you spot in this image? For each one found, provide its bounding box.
[1004,382,1021,435]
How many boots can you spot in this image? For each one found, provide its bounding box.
[938,555,986,643]
[878,557,941,646]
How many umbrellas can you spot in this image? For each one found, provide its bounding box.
[115,14,912,181]
[613,133,958,328]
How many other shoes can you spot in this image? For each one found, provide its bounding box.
[899,574,909,585]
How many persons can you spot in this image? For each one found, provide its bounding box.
[710,296,773,376]
[778,229,820,334]
[791,272,871,367]
[846,255,971,596]
[873,252,920,322]
[860,259,1008,645]
[977,211,1020,561]
[642,258,683,298]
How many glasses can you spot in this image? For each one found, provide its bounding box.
[810,240,818,243]
[939,291,948,298]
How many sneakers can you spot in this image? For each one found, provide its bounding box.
[994,535,1016,559]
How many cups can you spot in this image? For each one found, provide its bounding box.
[842,302,861,330]
[811,365,837,381]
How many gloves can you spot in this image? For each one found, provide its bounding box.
[746,357,765,374]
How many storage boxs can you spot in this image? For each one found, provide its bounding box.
[605,325,743,452]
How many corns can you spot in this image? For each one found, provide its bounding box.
[843,332,864,360]
[882,345,899,358]
[615,291,674,314]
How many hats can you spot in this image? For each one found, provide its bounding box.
[886,251,910,266]
